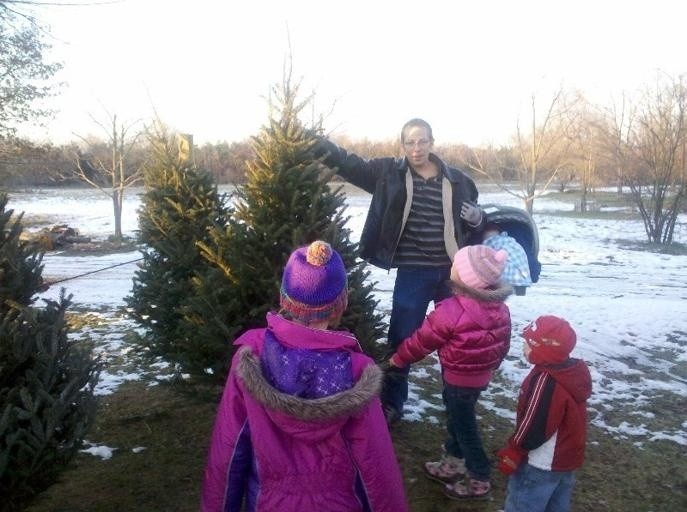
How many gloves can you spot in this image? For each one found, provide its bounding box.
[494,445,524,476]
[458,200,482,225]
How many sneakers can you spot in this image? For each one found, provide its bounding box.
[381,404,401,431]
[443,479,491,501]
[424,457,466,485]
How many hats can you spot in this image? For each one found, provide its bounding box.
[518,315,577,362]
[453,246,506,290]
[279,240,348,323]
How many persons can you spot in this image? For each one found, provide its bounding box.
[388,244,516,501]
[493,315,593,511]
[200,239,409,512]
[301,115,488,429]
